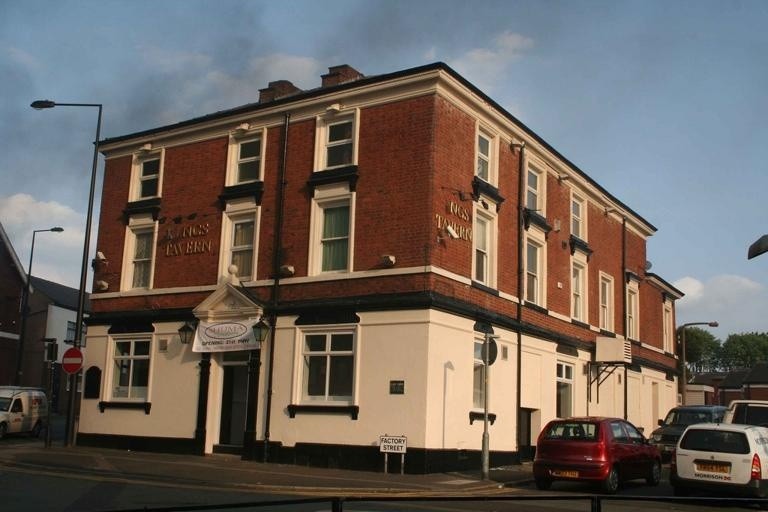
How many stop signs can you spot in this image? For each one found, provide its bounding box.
[61,346,84,375]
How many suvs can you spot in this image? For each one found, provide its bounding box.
[650,404,728,464]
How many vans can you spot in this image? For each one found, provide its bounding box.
[532,416,662,495]
[669,421,768,510]
[0,385,49,440]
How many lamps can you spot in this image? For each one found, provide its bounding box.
[174,316,195,342]
[249,311,276,346]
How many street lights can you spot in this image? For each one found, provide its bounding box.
[30,101,102,447]
[747,234,767,259]
[681,321,719,406]
[40,338,57,447]
[15,227,64,386]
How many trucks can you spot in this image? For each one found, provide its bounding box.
[722,399,768,428]
